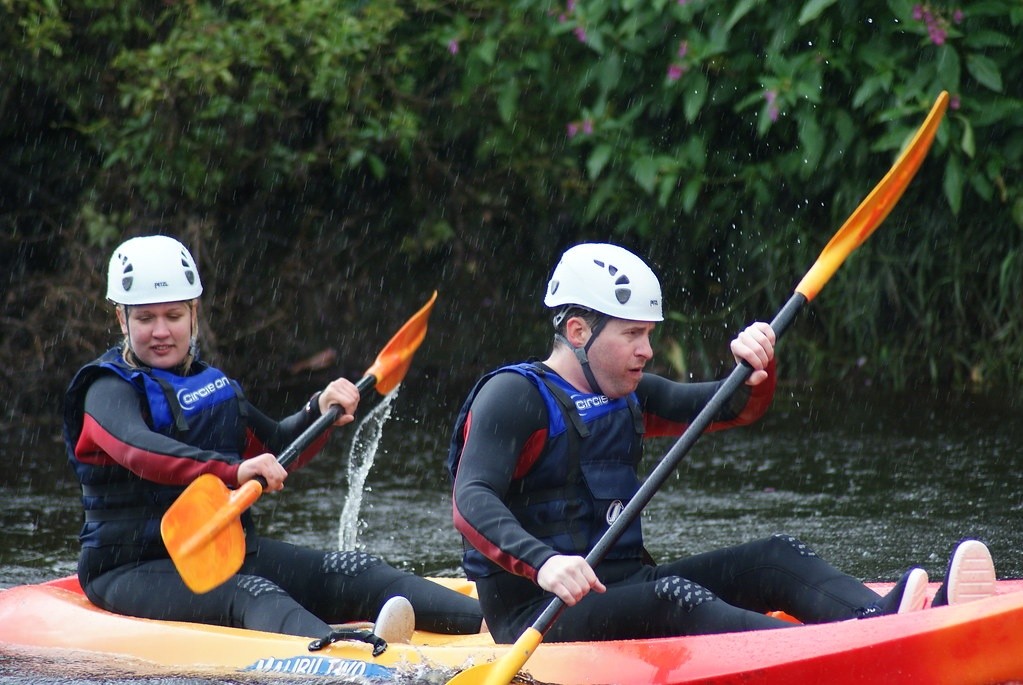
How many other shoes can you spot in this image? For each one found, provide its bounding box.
[931,538,996,609]
[371,594,416,644]
[857,567,928,618]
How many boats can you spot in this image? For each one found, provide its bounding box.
[0,578,1023,685]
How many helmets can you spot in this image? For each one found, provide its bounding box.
[544,243,664,322]
[106,233,203,305]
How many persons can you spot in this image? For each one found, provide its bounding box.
[453,243,996,645]
[62,236,488,645]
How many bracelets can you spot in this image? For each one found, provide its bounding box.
[310,392,322,419]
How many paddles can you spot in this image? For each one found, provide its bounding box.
[434,87,950,685]
[156,288,442,596]
